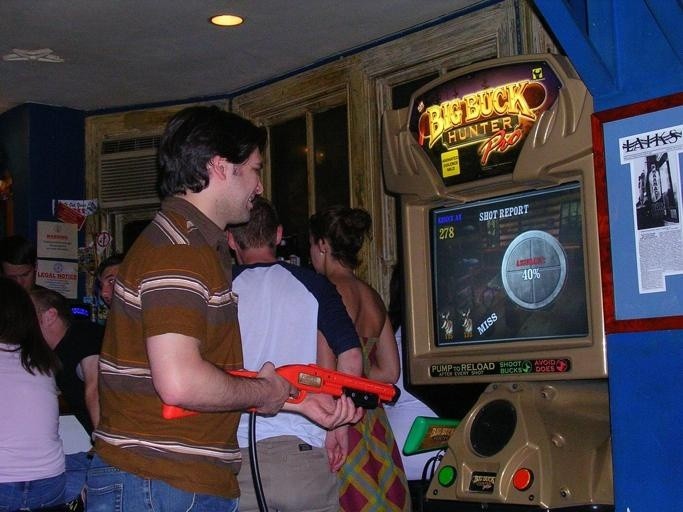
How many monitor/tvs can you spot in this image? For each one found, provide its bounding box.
[433,183,588,346]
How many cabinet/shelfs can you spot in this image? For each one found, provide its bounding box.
[82,97,230,266]
[229,1,594,323]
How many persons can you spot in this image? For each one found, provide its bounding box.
[1,236,128,511]
[309,205,412,511]
[225,194,363,511]
[86,105,363,512]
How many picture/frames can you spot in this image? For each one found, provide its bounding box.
[591,96,683,333]
